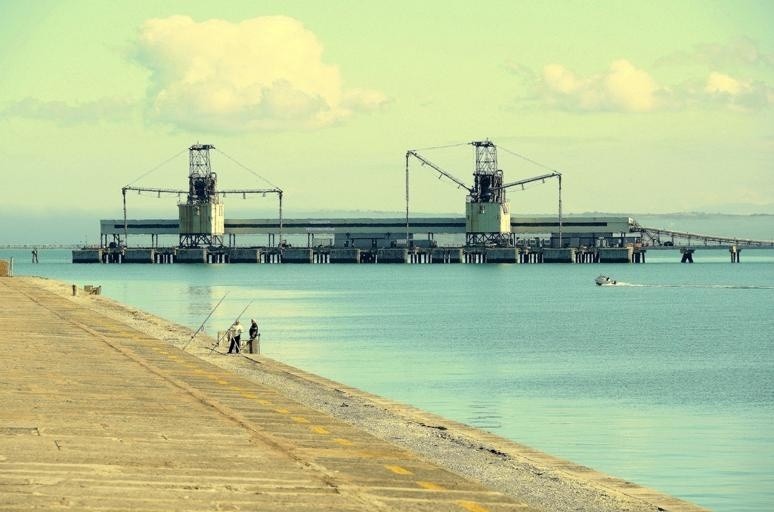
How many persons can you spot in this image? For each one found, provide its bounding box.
[246,318,259,354]
[227,319,243,355]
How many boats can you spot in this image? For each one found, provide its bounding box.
[594,274,617,286]
